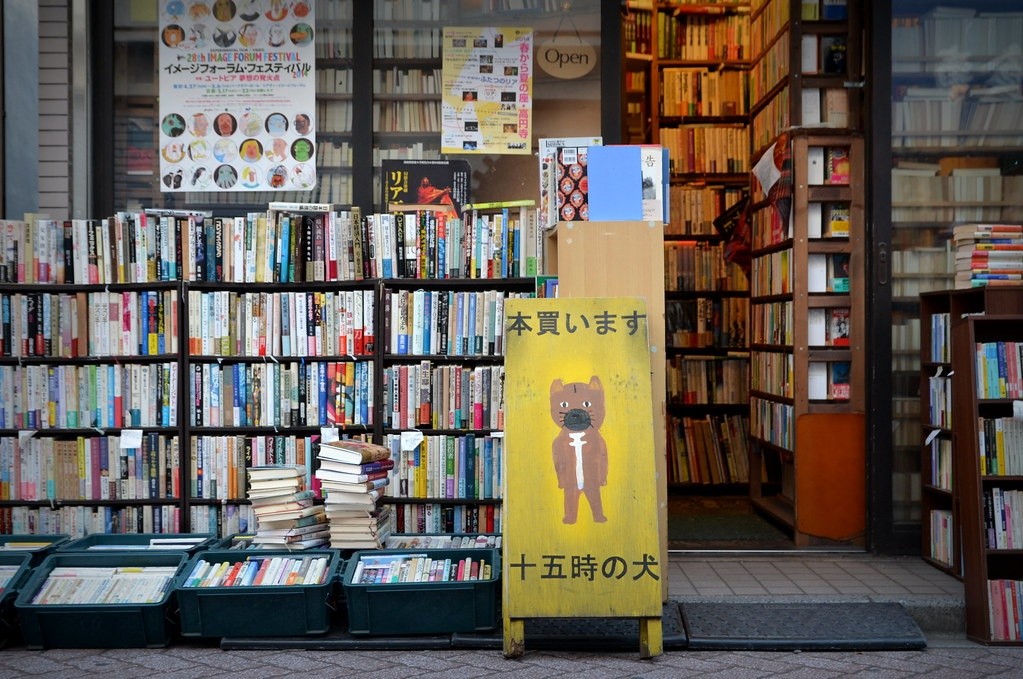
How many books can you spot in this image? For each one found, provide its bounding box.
[658,124,853,295]
[976,342,1023,647]
[0,200,545,283]
[664,297,851,347]
[0,290,536,356]
[349,534,502,584]
[0,436,504,500]
[314,440,393,550]
[667,350,853,486]
[952,224,1023,289]
[478,1,852,130]
[1,363,504,428]
[890,6,1023,225]
[0,504,504,542]
[893,247,952,526]
[926,312,964,580]
[182,536,329,585]
[242,465,331,550]
[112,0,444,218]
[0,537,207,604]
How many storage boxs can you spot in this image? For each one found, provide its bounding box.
[0,534,503,650]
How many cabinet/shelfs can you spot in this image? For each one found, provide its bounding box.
[625,1,1023,647]
[0,0,624,538]
